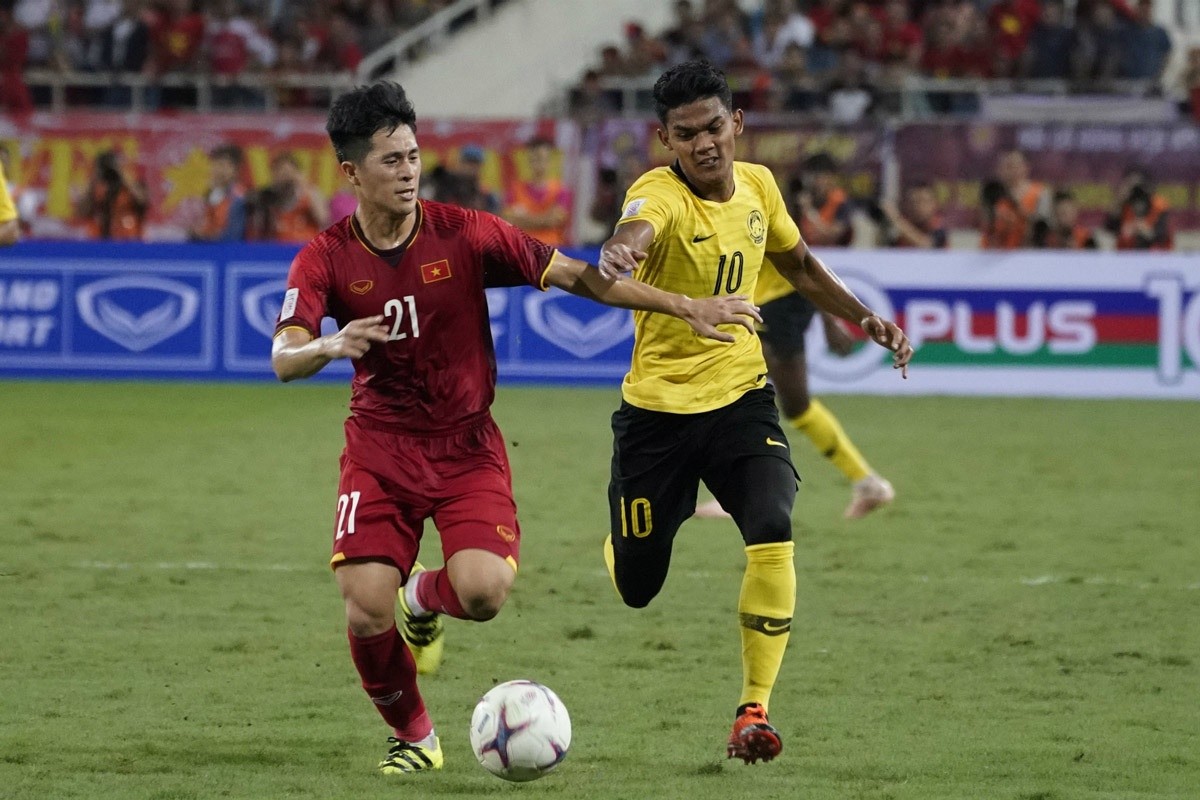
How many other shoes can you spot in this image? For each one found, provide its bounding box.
[844,475,896,520]
[693,501,732,518]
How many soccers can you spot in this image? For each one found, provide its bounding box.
[469,679,574,784]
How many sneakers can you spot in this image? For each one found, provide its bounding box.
[727,703,783,766]
[396,559,445,677]
[377,735,444,776]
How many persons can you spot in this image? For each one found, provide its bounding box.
[0,0,1199,248]
[694,256,898,521]
[597,60,915,766]
[270,78,765,775]
